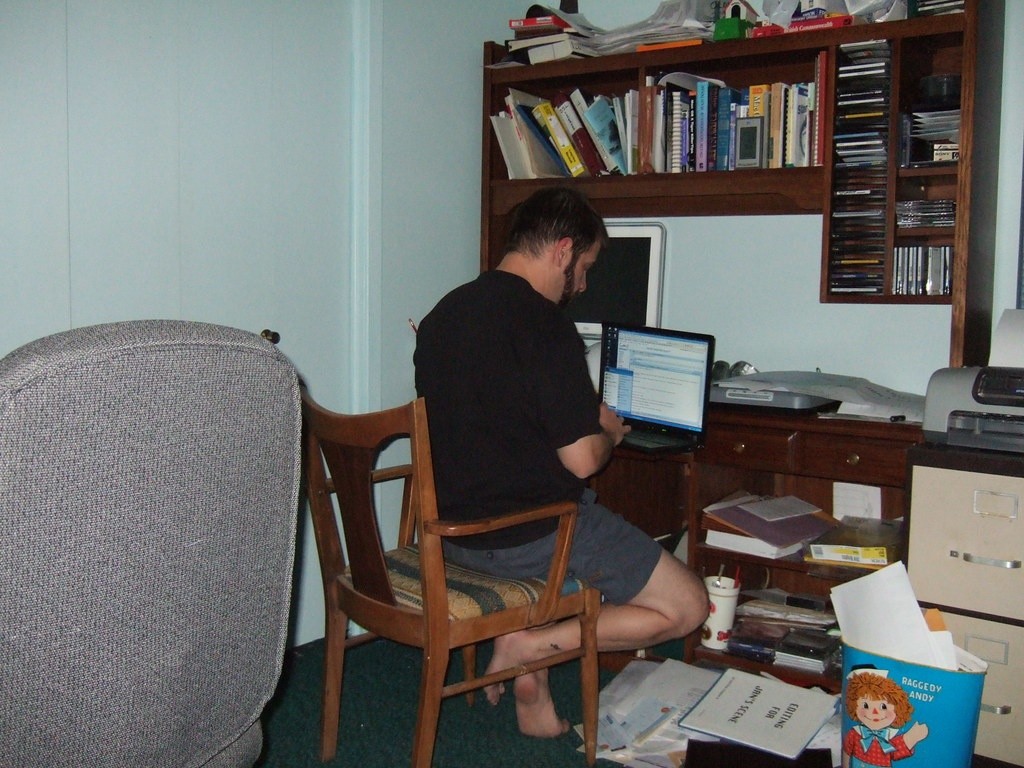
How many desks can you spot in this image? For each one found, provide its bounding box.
[588,445,694,573]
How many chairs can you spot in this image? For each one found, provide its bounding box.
[302,377,599,768]
[0,317,303,768]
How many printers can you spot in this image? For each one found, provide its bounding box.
[922,365,1024,455]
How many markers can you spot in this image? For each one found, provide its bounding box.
[890,415,906,422]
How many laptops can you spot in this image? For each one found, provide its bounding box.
[596,321,715,453]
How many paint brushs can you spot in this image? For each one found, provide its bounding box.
[407,318,418,333]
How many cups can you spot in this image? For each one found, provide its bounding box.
[700,576,741,651]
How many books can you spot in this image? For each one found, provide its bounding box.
[913,0,965,17]
[726,587,844,675]
[574,656,844,768]
[895,197,957,230]
[502,3,604,66]
[585,1,717,58]
[747,12,864,41]
[699,489,907,586]
[899,107,961,169]
[892,246,955,295]
[491,83,640,176]
[651,76,815,173]
[827,36,889,294]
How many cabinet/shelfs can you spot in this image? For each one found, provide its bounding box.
[682,402,926,695]
[480,20,828,218]
[819,12,1006,305]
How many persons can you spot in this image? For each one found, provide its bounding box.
[413,190,710,737]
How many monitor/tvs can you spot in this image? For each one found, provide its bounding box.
[565,222,667,340]
